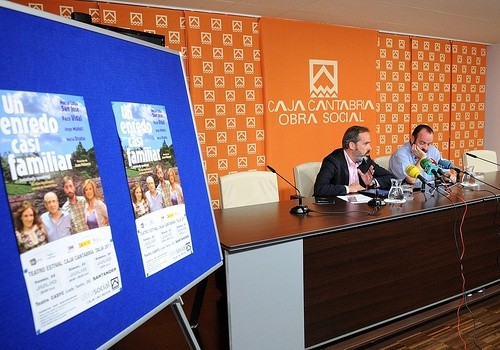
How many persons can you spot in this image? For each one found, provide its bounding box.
[167,168,184,206]
[61,176,89,236]
[154,165,173,207]
[14,201,48,254]
[314,126,395,197]
[39,192,73,242]
[132,183,150,220]
[144,176,164,212]
[82,179,109,230]
[388,125,456,185]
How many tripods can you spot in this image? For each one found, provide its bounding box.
[412,175,467,201]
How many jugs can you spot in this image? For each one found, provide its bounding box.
[462,166,477,188]
[388,179,404,202]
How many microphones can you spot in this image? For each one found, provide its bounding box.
[438,158,469,176]
[405,165,429,186]
[466,153,500,166]
[266,165,309,214]
[421,160,440,179]
[363,157,386,205]
[427,157,445,175]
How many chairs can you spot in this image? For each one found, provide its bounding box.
[294,162,322,198]
[463,150,497,173]
[218,171,279,209]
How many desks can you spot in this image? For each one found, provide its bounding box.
[197,171,500,350]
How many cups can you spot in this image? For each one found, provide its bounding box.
[474,171,485,186]
[402,185,414,201]
